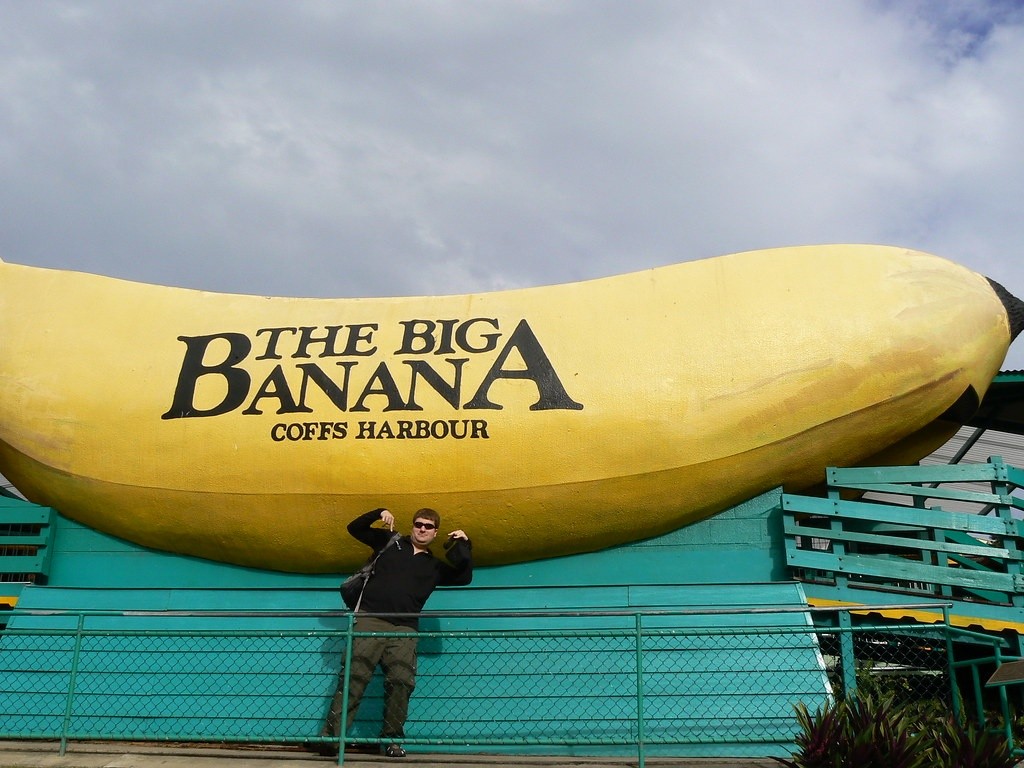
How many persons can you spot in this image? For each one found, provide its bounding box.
[304,508,472,756]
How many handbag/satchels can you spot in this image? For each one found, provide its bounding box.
[340,531,402,610]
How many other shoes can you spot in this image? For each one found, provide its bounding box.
[303,742,337,756]
[386,743,406,757]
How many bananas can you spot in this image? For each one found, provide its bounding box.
[0,244,1024,571]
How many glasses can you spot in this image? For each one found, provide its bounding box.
[414,522,436,529]
